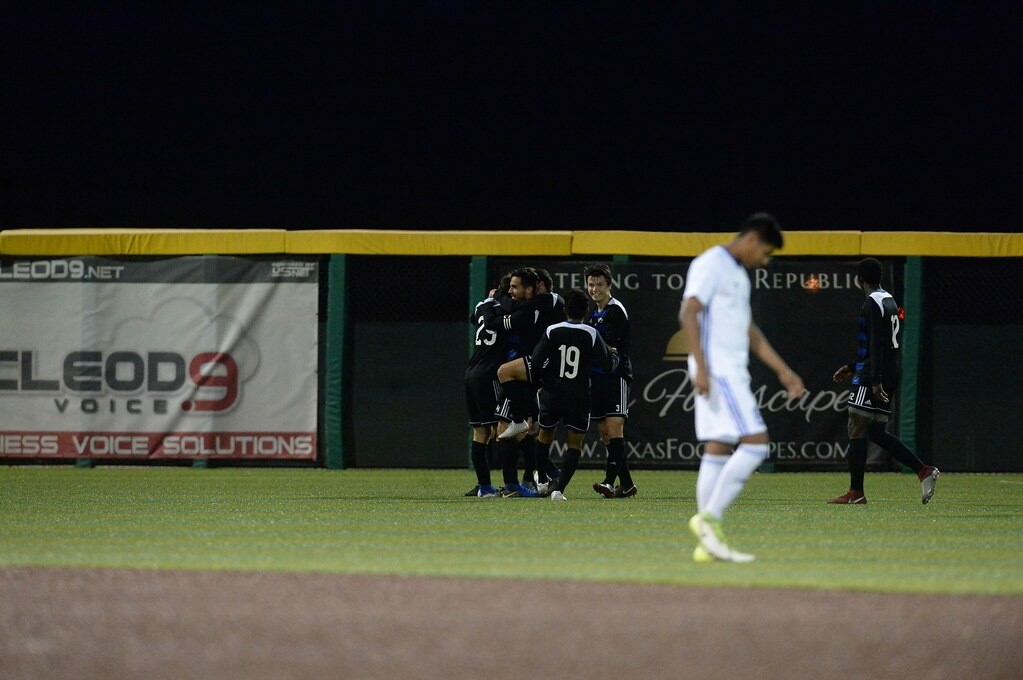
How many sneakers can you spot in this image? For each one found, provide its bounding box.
[503,482,538,499]
[827,492,867,504]
[478,486,502,498]
[616,484,637,498]
[692,544,754,563]
[920,466,940,504]
[593,483,615,498]
[688,515,729,561]
[520,471,566,500]
[498,419,530,440]
[465,485,479,496]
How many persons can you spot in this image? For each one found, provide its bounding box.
[464,265,638,501]
[679,214,804,563]
[828,257,939,504]
